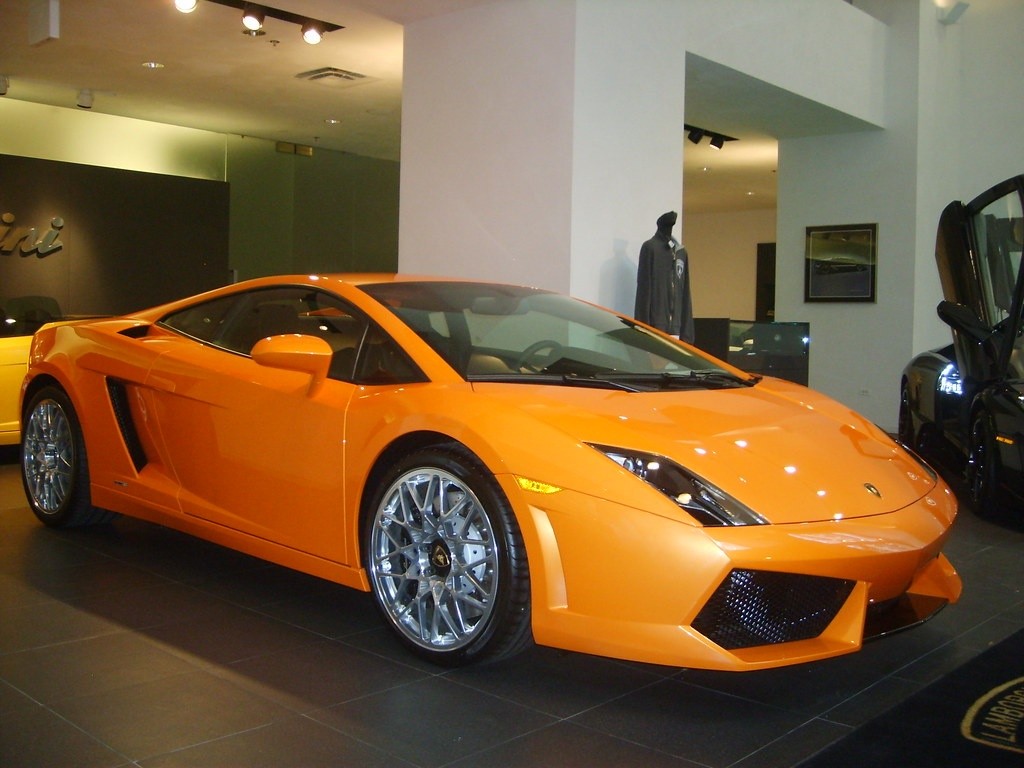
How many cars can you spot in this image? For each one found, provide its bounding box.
[0,312,115,446]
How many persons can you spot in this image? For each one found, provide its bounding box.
[635,210,695,345]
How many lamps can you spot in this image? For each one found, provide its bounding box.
[301,21,323,44]
[709,137,723,150]
[241,8,265,30]
[688,131,702,144]
[175,0,198,13]
[76,89,92,110]
[0,75,7,95]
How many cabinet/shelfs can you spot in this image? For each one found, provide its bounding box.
[694,318,810,387]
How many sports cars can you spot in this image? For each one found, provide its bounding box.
[15,271,962,680]
[896,176,1024,521]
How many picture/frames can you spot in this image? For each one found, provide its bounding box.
[804,223,879,303]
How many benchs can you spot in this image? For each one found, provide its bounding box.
[214,303,383,356]
